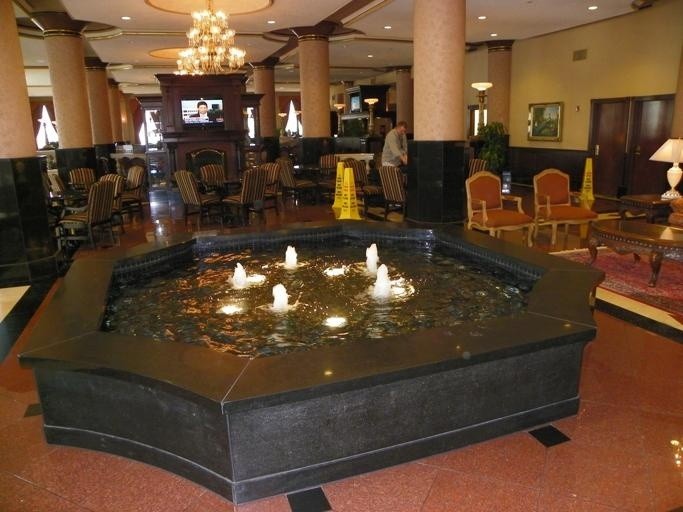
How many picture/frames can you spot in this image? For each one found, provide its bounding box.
[526,100,563,140]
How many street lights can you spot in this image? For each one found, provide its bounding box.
[471,79,493,137]
[362,98,379,135]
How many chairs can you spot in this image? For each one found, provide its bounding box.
[42,153,150,229]
[171,160,283,229]
[463,171,534,249]
[532,169,598,245]
[276,152,486,224]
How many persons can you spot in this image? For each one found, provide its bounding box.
[381,121,409,167]
[189,102,216,122]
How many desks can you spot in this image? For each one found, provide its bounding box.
[583,216,681,290]
[617,192,670,259]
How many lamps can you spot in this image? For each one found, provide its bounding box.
[469,81,493,130]
[650,135,682,201]
[363,97,378,131]
[169,0,245,78]
[278,112,287,129]
[294,110,300,135]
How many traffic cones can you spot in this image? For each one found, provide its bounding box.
[578,156,596,202]
[334,167,361,221]
[579,200,593,238]
[330,161,345,209]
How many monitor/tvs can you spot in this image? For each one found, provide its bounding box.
[350,92,360,112]
[180,95,225,129]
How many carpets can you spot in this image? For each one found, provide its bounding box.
[546,239,682,348]
